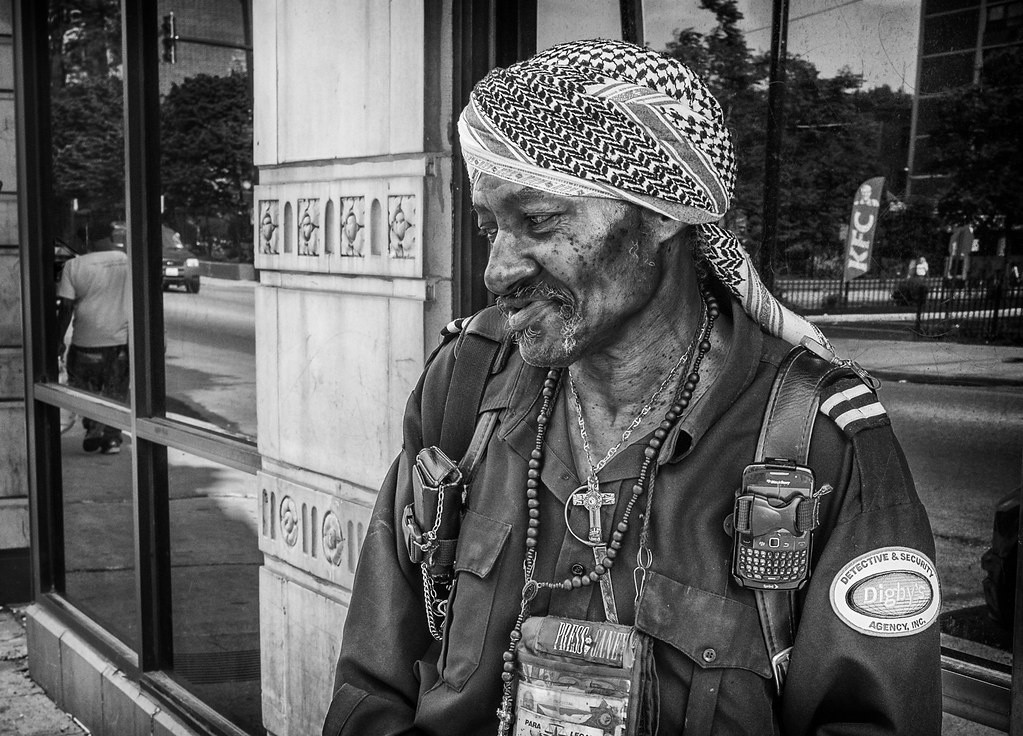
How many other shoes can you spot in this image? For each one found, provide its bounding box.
[80,435,122,454]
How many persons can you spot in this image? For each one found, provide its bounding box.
[51,216,132,455]
[321,38,944,736]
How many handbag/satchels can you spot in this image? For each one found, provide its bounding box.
[510,613,651,736]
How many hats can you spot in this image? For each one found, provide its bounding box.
[456,41,832,354]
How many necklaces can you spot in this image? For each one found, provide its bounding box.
[495,253,720,736]
[563,293,712,546]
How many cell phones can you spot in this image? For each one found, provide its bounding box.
[730,463,816,592]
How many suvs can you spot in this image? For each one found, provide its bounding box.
[106,221,201,293]
